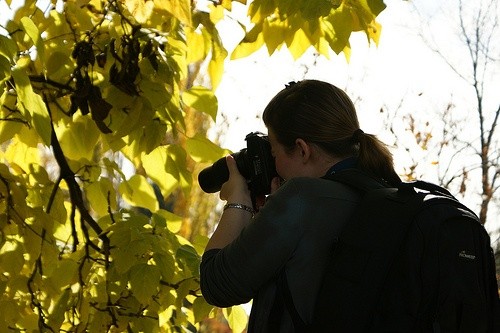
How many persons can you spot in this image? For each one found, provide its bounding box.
[200,78,412,332]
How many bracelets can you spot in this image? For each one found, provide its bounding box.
[223,203,254,218]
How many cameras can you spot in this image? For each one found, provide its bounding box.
[198,130,283,197]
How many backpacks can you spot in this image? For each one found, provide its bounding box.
[321,169,500,333]
